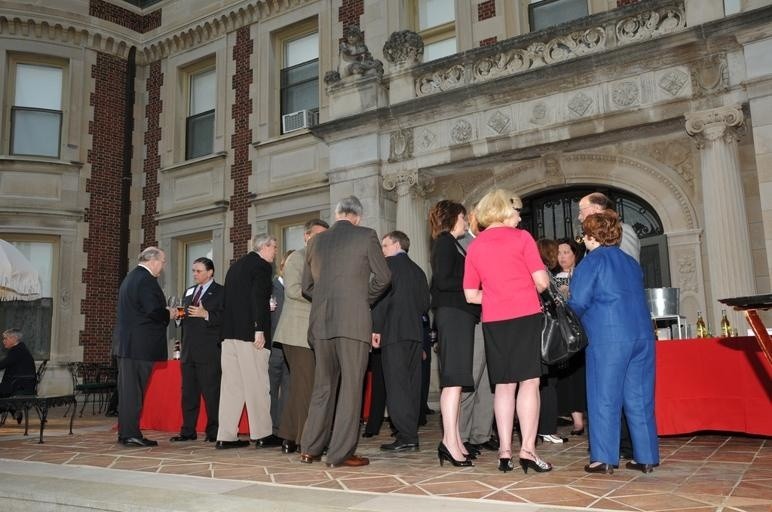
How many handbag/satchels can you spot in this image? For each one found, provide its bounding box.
[540,281,589,365]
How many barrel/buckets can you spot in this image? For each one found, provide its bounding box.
[643,286,681,319]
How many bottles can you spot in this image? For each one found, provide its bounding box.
[173,337,182,361]
[720,308,731,338]
[695,310,707,338]
[706,320,713,339]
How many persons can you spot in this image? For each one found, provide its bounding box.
[558,210,662,474]
[1,325,39,425]
[267,220,428,453]
[301,196,392,468]
[110,246,180,449]
[211,234,288,450]
[580,193,642,460]
[169,256,224,445]
[429,200,480,467]
[457,202,587,455]
[463,188,552,475]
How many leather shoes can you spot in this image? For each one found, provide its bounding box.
[169,434,197,441]
[380,440,418,452]
[125,438,158,447]
[626,462,653,472]
[584,463,613,474]
[327,455,369,466]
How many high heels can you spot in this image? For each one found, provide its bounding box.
[438,427,585,473]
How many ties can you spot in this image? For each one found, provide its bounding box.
[192,286,202,306]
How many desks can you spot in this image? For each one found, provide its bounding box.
[655,336,772,438]
[112,359,372,435]
[1,395,77,445]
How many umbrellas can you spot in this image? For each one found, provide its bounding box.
[0,238,43,305]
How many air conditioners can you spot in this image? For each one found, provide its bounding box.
[281,108,316,133]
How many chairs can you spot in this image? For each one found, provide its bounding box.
[2,360,49,423]
[62,360,114,416]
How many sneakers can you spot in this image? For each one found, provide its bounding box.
[205,435,312,463]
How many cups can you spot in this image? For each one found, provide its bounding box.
[167,295,177,312]
[726,327,739,337]
[269,294,277,312]
[176,298,185,321]
